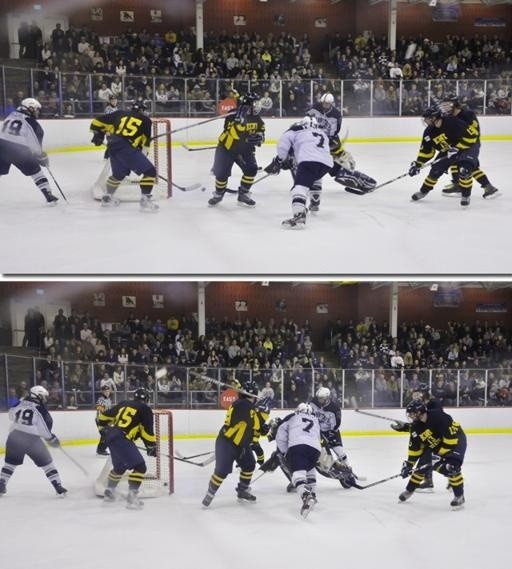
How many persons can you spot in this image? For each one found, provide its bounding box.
[102,95,120,140]
[0,305,511,412]
[207,92,266,208]
[0,21,512,120]
[202,381,271,508]
[397,401,469,507]
[236,418,274,468]
[1,385,70,501]
[407,105,481,208]
[268,417,356,492]
[438,93,503,199]
[97,389,158,508]
[94,384,113,456]
[274,402,324,517]
[308,385,349,467]
[304,91,356,171]
[0,97,60,208]
[263,117,335,228]
[390,388,443,492]
[89,102,162,212]
[329,159,376,194]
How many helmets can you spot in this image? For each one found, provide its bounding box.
[134,389,150,404]
[131,98,148,110]
[316,387,330,400]
[21,98,42,114]
[240,385,257,398]
[295,402,313,415]
[30,385,50,400]
[422,94,459,130]
[406,400,426,418]
[243,92,262,116]
[409,383,430,396]
[320,93,335,106]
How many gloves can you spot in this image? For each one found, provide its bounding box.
[409,161,420,177]
[146,442,156,456]
[264,155,293,174]
[401,461,413,478]
[34,152,49,166]
[91,132,104,146]
[46,434,60,448]
[445,449,463,465]
[391,420,410,432]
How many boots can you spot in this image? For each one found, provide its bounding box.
[300,492,317,515]
[42,188,58,202]
[209,190,225,204]
[283,212,306,226]
[238,187,255,205]
[140,194,159,209]
[52,480,67,494]
[309,198,320,212]
[417,477,434,488]
[101,194,120,206]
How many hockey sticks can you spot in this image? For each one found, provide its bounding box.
[322,434,430,489]
[136,448,215,467]
[351,396,398,422]
[224,174,269,194]
[181,144,217,151]
[345,156,447,195]
[156,174,202,191]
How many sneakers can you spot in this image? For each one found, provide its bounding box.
[460,196,471,205]
[202,492,214,506]
[442,186,462,193]
[104,487,114,500]
[411,192,426,200]
[451,494,465,506]
[128,492,144,506]
[237,490,256,501]
[483,186,497,198]
[399,490,414,500]
